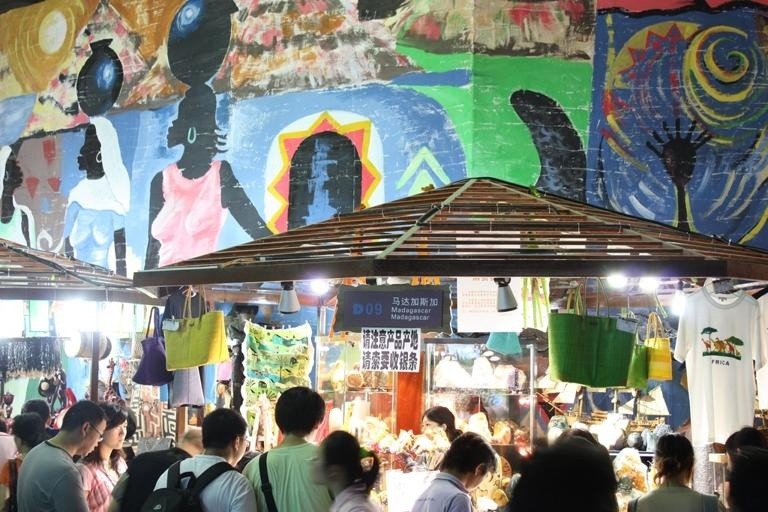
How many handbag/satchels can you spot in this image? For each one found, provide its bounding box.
[132,307,174,386]
[3,458,19,512]
[547,278,673,389]
[162,285,230,372]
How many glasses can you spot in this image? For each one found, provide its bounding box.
[89,424,105,444]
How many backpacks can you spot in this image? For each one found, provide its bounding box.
[140,458,240,511]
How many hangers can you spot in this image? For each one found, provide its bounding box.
[182,284,197,298]
[704,277,742,299]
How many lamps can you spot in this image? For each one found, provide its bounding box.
[278,281,301,315]
[496,279,517,312]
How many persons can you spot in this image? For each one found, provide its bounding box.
[0,387,768,511]
